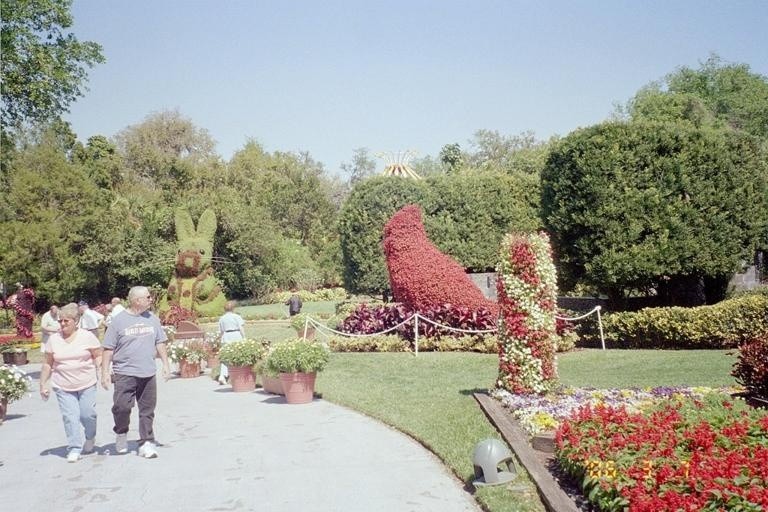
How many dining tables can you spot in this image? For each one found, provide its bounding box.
[0,398,8,421]
[1,347,30,366]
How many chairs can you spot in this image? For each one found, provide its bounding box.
[115,436,128,453]
[66,452,79,462]
[84,440,94,452]
[138,440,158,458]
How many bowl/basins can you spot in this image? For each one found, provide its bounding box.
[63,333,75,343]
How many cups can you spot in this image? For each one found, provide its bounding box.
[58,320,69,322]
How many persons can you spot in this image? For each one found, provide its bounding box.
[40,303,102,464]
[285,288,303,314]
[102,285,170,458]
[219,300,247,385]
[41,297,124,367]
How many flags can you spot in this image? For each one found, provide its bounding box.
[297,329,316,340]
[261,369,284,394]
[282,370,316,404]
[218,339,262,365]
[228,366,257,391]
[178,358,199,377]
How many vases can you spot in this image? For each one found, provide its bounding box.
[262,338,329,373]
[0,364,34,402]
[289,311,320,329]
[165,342,209,364]
